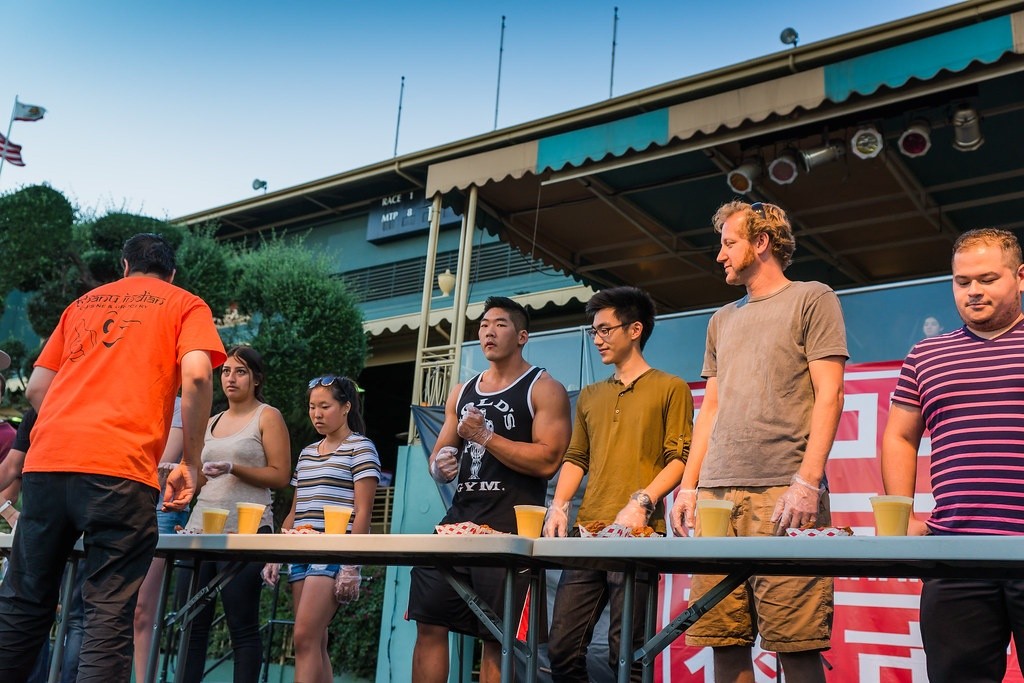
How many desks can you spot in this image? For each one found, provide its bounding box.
[533,536,1024,683]
[0,534,533,683]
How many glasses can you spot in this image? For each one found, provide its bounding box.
[587,322,635,337]
[309,376,350,399]
[750,201,766,219]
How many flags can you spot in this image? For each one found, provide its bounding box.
[0,132,26,166]
[13,100,46,121]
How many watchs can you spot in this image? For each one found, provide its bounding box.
[631,493,653,514]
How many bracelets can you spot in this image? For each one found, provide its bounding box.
[0,500,12,515]
[221,462,233,474]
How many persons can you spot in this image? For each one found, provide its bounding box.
[923,313,944,337]
[404,295,571,683]
[670,197,849,683]
[0,233,381,683]
[881,225,1024,683]
[543,286,695,683]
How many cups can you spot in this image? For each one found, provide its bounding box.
[868,496,914,535]
[696,499,734,537]
[201,506,230,534]
[322,506,354,535]
[235,501,266,534]
[513,505,548,540]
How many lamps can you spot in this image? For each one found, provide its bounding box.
[796,126,846,176]
[727,146,765,195]
[252,179,267,195]
[897,116,932,158]
[851,123,883,160]
[948,100,986,153]
[779,27,798,49]
[767,140,799,185]
